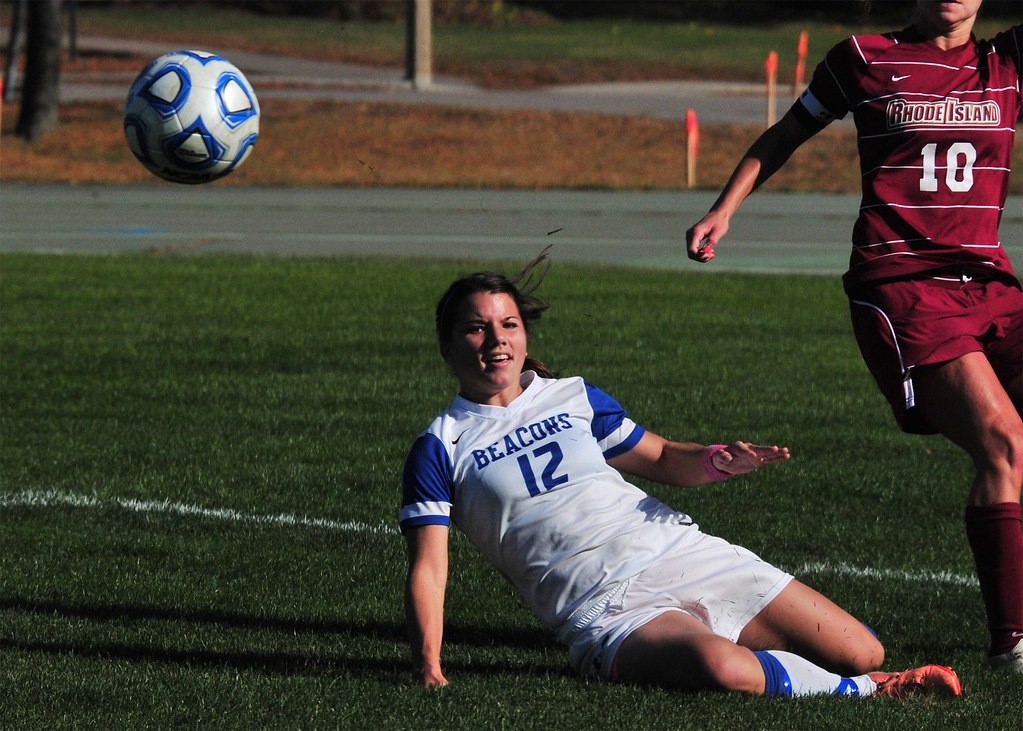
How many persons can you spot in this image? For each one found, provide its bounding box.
[686,0,1023,673]
[396,273,965,701]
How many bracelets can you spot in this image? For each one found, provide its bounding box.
[702,445,733,481]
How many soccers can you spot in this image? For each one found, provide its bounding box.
[123,49,262,185]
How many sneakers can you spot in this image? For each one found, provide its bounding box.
[986,638,1023,674]
[867,665,961,699]
